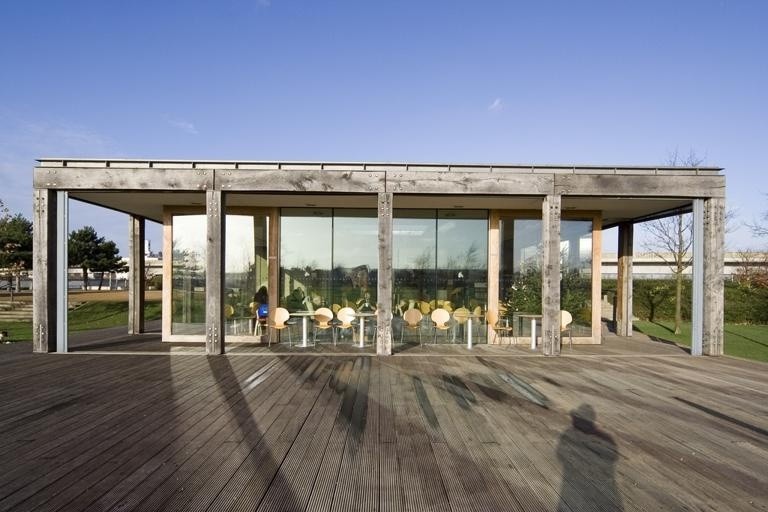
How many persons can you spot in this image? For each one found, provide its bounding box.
[251,286,267,336]
[0,331,8,344]
[355,292,377,344]
[284,286,307,311]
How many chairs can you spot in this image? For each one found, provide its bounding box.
[267,307,294,348]
[399,308,425,348]
[485,310,514,346]
[452,306,484,343]
[394,297,488,328]
[334,307,358,346]
[430,308,453,343]
[560,309,572,349]
[312,307,335,345]
[225,301,268,337]
[280,297,378,343]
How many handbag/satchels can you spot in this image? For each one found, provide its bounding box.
[258,304,268,317]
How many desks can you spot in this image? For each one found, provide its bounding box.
[517,314,543,350]
[456,313,484,350]
[288,313,318,348]
[347,313,377,348]
